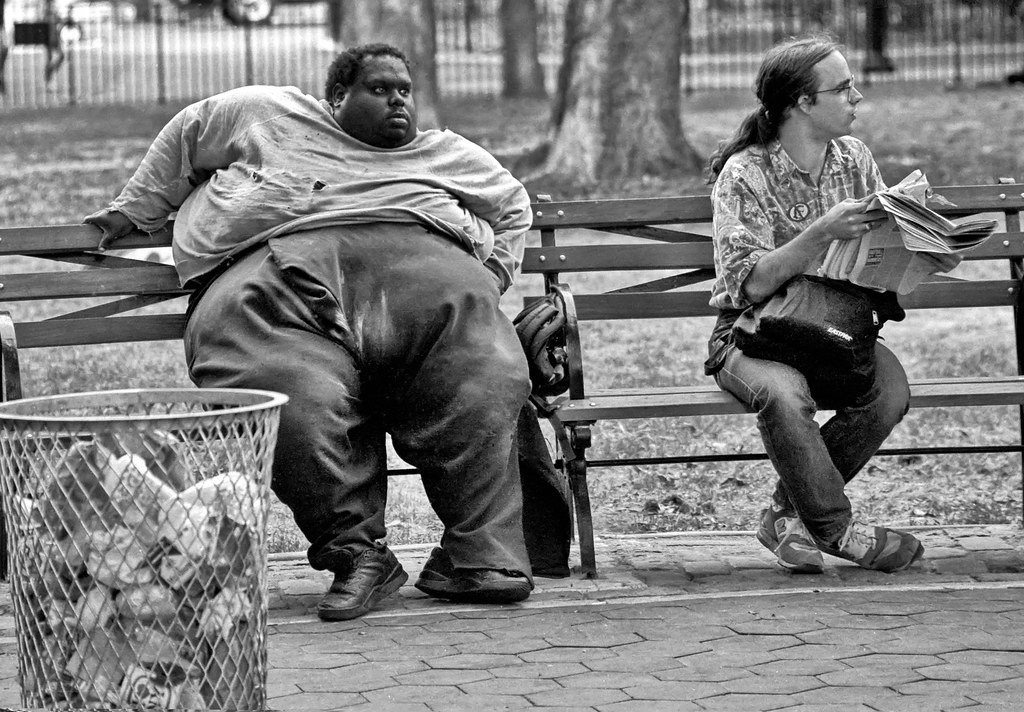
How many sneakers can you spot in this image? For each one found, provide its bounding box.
[814,518,923,573]
[318,541,409,620]
[414,546,530,603]
[757,502,825,572]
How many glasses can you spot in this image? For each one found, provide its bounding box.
[811,75,855,102]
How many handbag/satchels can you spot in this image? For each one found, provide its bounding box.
[512,290,571,395]
[731,270,906,397]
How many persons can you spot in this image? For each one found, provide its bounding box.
[82,42,533,620]
[705,39,963,574]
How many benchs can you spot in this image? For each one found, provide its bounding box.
[0,178,1024,576]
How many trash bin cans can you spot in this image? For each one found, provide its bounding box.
[0,388,290,712]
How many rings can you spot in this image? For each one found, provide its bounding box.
[867,223,872,231]
